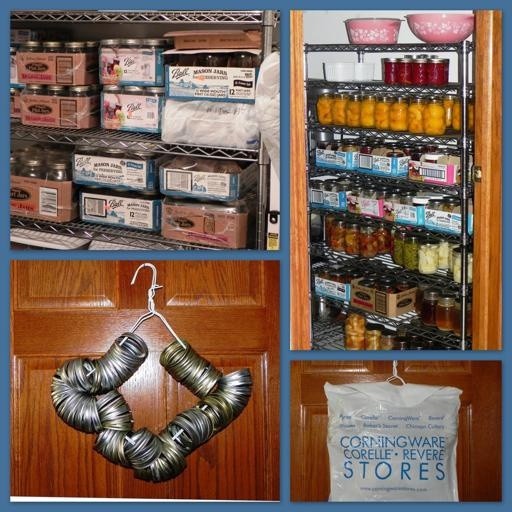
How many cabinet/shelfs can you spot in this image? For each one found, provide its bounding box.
[305,39,475,351]
[9,8,276,251]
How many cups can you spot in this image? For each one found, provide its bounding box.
[175,54,263,69]
[324,61,377,83]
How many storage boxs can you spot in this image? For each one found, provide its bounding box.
[309,141,471,320]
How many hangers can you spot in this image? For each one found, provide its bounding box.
[86,258,223,455]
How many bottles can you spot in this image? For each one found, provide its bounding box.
[202,211,216,236]
[10,145,73,181]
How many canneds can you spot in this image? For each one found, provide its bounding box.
[316,57,475,350]
[11,37,262,214]
[203,214,216,235]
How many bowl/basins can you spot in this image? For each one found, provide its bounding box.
[344,18,402,43]
[403,12,473,45]
